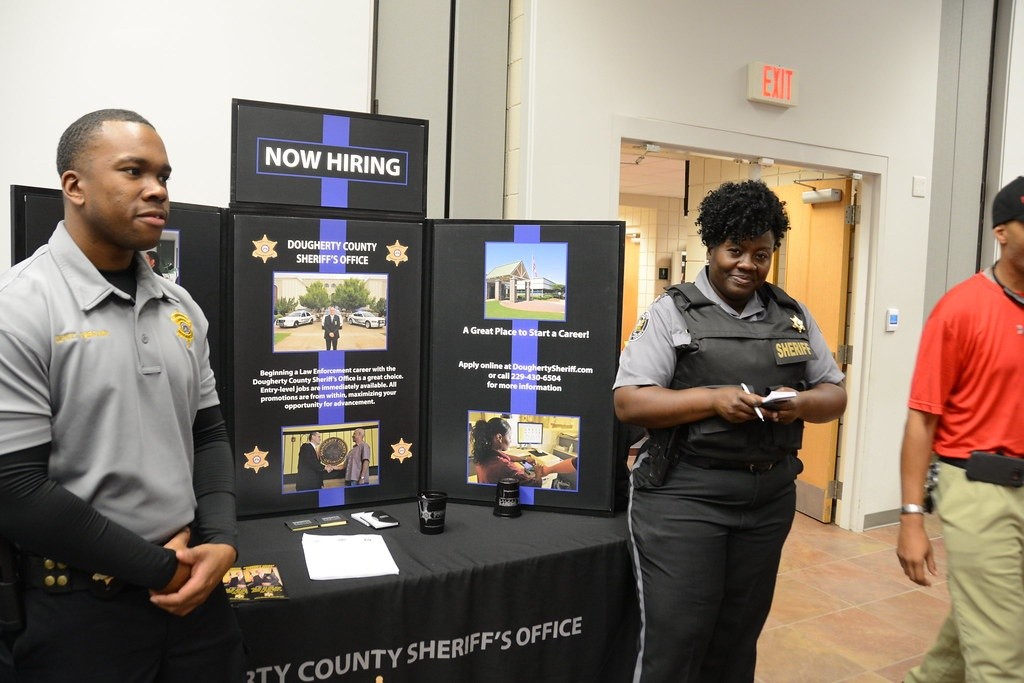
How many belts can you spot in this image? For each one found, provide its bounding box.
[648,444,798,474]
[10,551,122,594]
[938,453,969,470]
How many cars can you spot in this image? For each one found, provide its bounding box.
[321,310,343,329]
[348,312,386,328]
[277,310,313,328]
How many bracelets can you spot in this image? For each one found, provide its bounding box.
[899,504,923,516]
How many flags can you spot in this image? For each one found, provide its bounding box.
[533,256,537,277]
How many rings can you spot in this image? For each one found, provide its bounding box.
[772,412,779,422]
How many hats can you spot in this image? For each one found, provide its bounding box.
[993,176,1024,227]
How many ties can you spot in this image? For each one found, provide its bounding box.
[315,448,319,460]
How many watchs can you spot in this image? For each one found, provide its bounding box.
[360,476,365,479]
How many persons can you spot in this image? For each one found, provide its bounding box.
[324,307,340,351]
[145,251,163,278]
[473,418,578,488]
[296,429,370,491]
[610,178,847,683]
[0,109,251,683]
[897,175,1024,683]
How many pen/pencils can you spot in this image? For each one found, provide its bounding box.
[740,382,765,422]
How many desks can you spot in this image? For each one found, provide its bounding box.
[205,488,641,683]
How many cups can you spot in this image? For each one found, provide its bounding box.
[493,478,522,519]
[416,490,448,535]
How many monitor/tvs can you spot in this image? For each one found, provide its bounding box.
[518,422,543,445]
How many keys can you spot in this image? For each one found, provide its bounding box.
[924,465,938,513]
